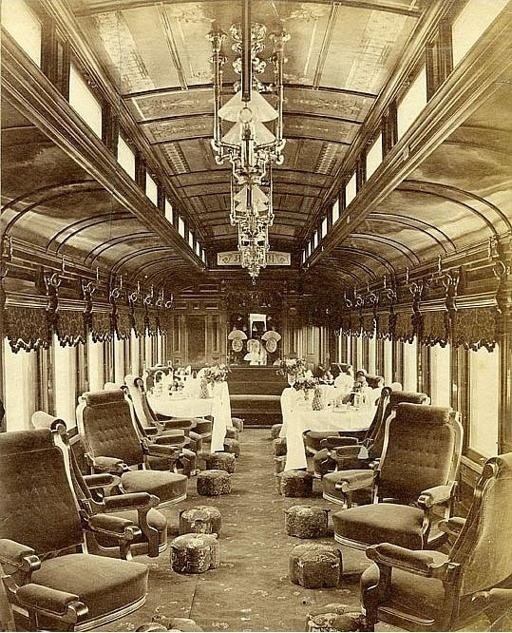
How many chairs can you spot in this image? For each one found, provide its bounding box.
[73,389,188,508]
[359,452,510,631]
[104,364,214,479]
[2,429,151,630]
[302,363,432,507]
[331,400,465,556]
[31,410,168,562]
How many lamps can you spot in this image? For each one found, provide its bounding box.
[204,0,292,287]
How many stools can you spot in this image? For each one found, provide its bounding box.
[305,601,364,632]
[175,416,244,497]
[179,505,222,536]
[289,542,345,589]
[136,614,206,633]
[282,505,329,540]
[169,533,217,575]
[270,422,314,498]
